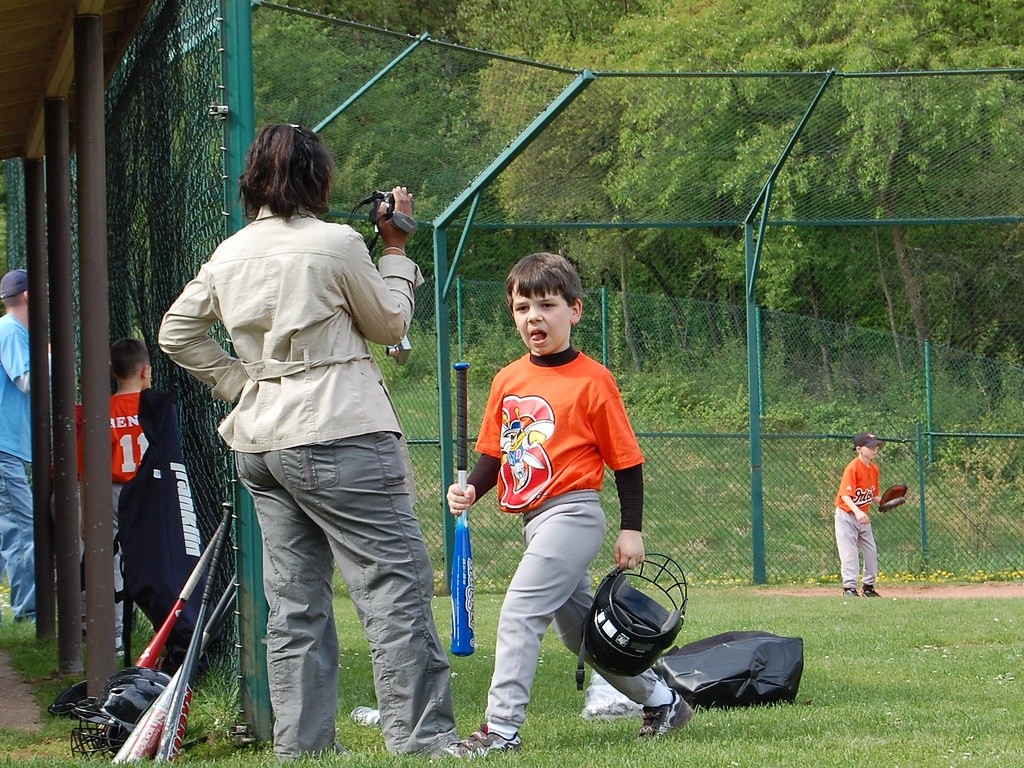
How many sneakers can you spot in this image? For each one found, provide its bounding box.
[442,724,522,760]
[637,689,693,737]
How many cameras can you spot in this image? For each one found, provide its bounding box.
[367,190,396,225]
[385,335,412,365]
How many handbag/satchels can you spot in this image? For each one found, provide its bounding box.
[654,630,803,712]
[112,388,211,668]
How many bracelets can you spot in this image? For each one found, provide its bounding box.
[385,247,405,256]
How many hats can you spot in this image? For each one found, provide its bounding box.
[0,270,28,298]
[854,433,882,448]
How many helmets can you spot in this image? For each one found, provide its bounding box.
[71,666,172,762]
[582,566,685,677]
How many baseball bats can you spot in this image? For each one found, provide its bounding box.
[447,360,476,658]
[109,575,239,767]
[95,501,234,768]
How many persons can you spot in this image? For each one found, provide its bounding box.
[76,338,151,657]
[835,432,882,599]
[0,269,51,627]
[431,253,693,763]
[158,123,461,763]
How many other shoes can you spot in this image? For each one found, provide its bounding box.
[862,591,881,597]
[843,589,860,597]
